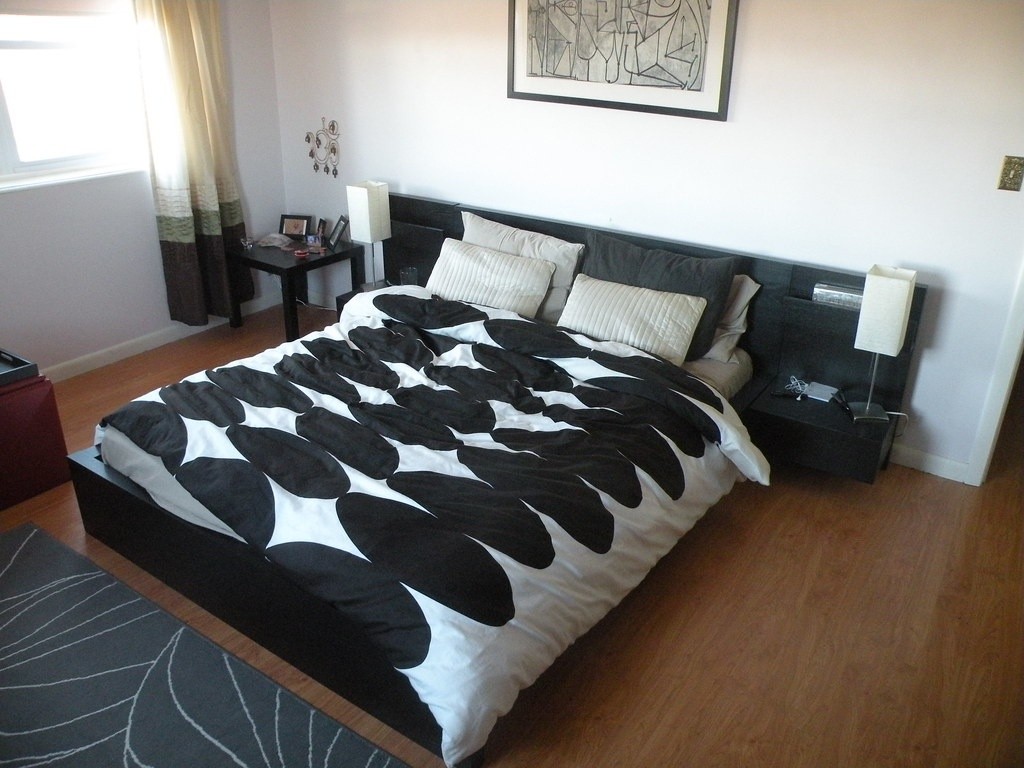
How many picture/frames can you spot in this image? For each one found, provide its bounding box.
[316,219,327,243]
[279,214,312,241]
[326,215,348,250]
[506,0,739,122]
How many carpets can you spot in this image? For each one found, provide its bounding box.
[1,520,417,768]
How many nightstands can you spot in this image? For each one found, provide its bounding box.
[747,375,900,484]
[335,278,389,324]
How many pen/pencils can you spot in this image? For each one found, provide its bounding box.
[830,389,855,424]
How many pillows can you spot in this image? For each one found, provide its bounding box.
[460,210,586,325]
[425,238,557,320]
[578,230,741,360]
[555,274,706,367]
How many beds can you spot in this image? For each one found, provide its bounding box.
[64,192,930,768]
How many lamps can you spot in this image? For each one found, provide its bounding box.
[347,179,391,293]
[703,272,760,364]
[846,263,918,425]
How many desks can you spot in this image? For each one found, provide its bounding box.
[223,233,364,342]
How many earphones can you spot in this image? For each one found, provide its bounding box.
[796,394,802,401]
[802,380,809,387]
